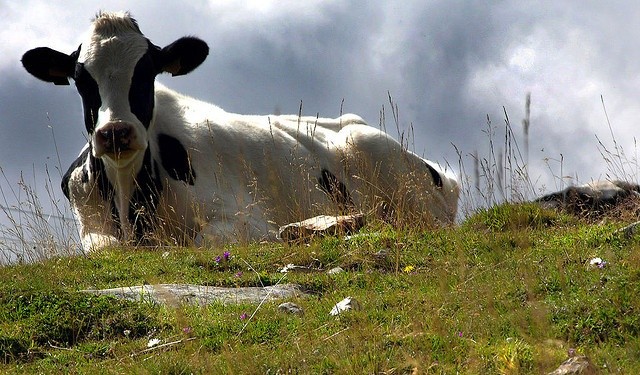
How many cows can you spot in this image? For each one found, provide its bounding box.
[20,9,461,256]
